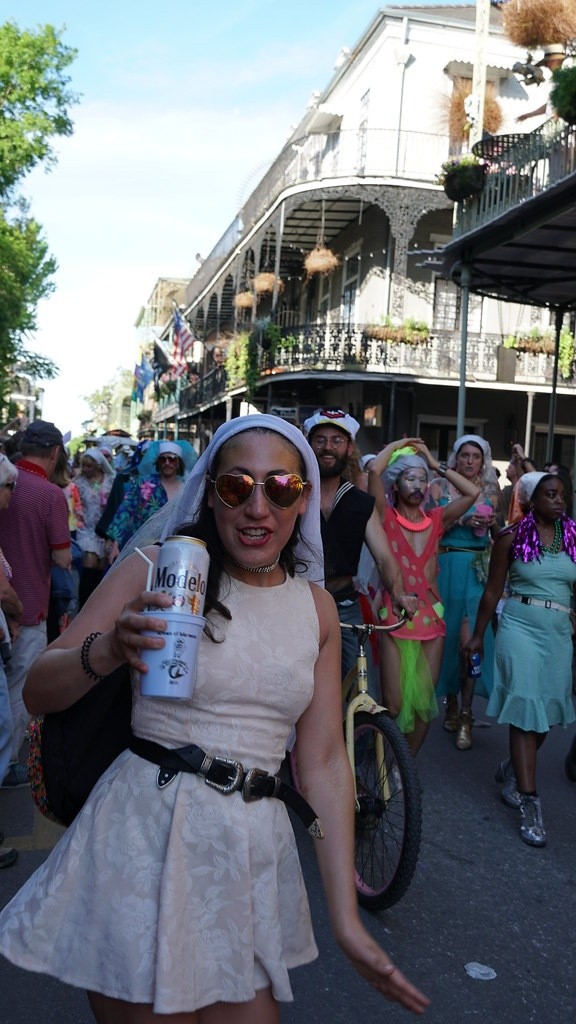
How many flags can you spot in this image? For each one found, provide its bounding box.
[132,366,144,403]
[172,307,197,381]
[140,355,156,389]
[152,339,183,402]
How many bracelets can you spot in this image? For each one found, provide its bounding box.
[82,631,106,682]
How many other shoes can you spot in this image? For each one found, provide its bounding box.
[457,712,472,751]
[0,846,18,869]
[2,763,34,789]
[443,698,458,733]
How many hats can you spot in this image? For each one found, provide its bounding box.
[24,420,68,459]
[157,442,182,459]
[302,408,360,445]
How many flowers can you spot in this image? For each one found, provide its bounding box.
[433,156,482,186]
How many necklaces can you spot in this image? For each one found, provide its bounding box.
[393,505,432,531]
[533,521,562,556]
[222,550,280,574]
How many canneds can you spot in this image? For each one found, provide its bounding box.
[467,651,481,677]
[147,536,209,618]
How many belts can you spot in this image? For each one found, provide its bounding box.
[511,592,571,614]
[129,733,324,840]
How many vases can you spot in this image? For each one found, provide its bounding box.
[446,167,488,203]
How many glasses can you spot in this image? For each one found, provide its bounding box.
[0,482,16,491]
[312,437,349,449]
[157,456,178,465]
[210,473,308,510]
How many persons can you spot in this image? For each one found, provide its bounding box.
[0,412,432,1024]
[515,44,576,177]
[0,410,576,868]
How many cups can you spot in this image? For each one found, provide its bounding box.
[473,504,492,537]
[138,611,206,701]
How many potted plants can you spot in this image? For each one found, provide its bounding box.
[503,324,576,379]
[254,318,287,374]
[496,335,519,382]
[365,315,437,350]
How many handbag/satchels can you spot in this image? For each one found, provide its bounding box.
[26,663,132,824]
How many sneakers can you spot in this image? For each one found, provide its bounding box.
[495,760,521,808]
[520,793,547,846]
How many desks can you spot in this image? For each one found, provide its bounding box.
[471,132,557,204]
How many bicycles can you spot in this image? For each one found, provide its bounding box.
[332,593,423,913]
